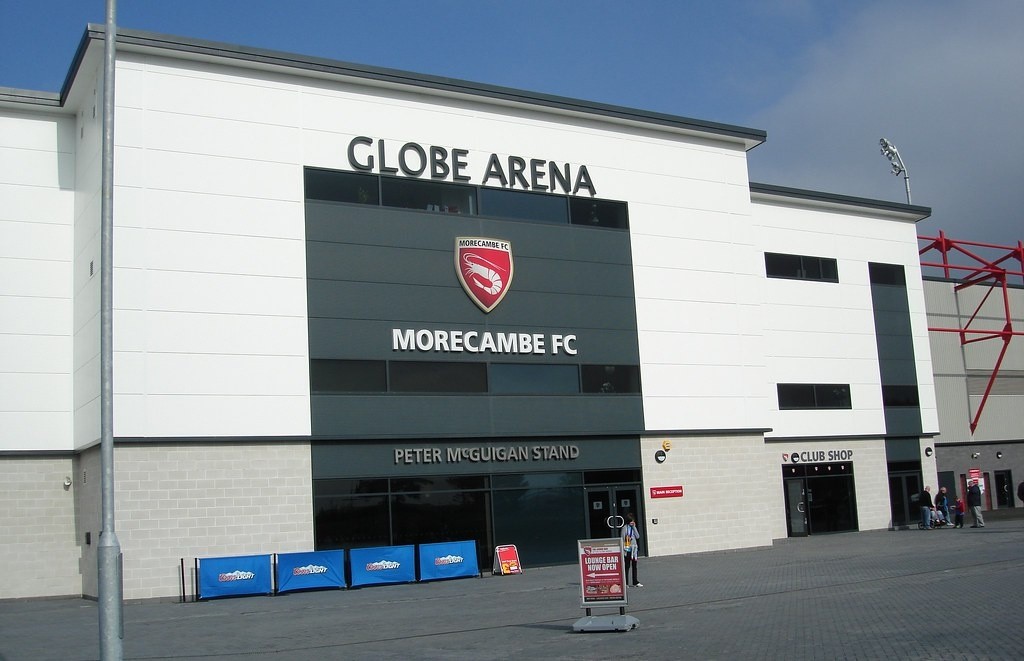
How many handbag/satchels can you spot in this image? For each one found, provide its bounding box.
[625,524,630,547]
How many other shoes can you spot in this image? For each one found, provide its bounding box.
[981,526,984,527]
[626,585,629,588]
[942,520,945,522]
[924,528,927,529]
[927,527,933,529]
[947,522,954,526]
[634,583,643,587]
[970,525,977,528]
[936,520,939,522]
[953,525,959,528]
[960,526,964,528]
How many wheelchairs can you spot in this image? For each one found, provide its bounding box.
[918,507,947,530]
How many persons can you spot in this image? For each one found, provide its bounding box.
[621,513,643,588]
[967,481,985,528]
[935,487,954,526]
[919,486,945,529]
[953,496,964,529]
